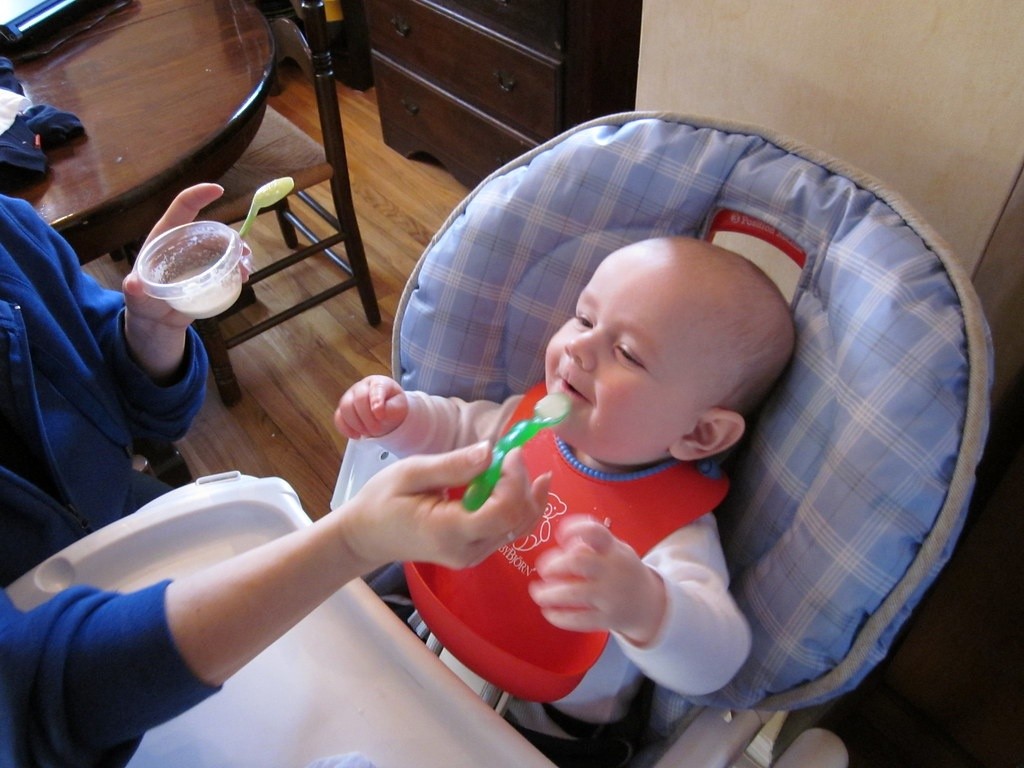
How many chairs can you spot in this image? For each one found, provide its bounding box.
[119,0,380,408]
[334,107,996,768]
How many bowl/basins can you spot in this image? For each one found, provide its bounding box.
[136,220,243,319]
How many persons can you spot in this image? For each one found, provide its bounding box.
[333,236,796,768]
[2,184,555,768]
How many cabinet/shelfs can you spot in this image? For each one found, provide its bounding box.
[365,0,645,193]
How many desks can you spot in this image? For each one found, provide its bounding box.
[2,472,565,767]
[1,0,274,403]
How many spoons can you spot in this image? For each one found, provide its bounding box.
[237,176,294,239]
[461,393,572,511]
